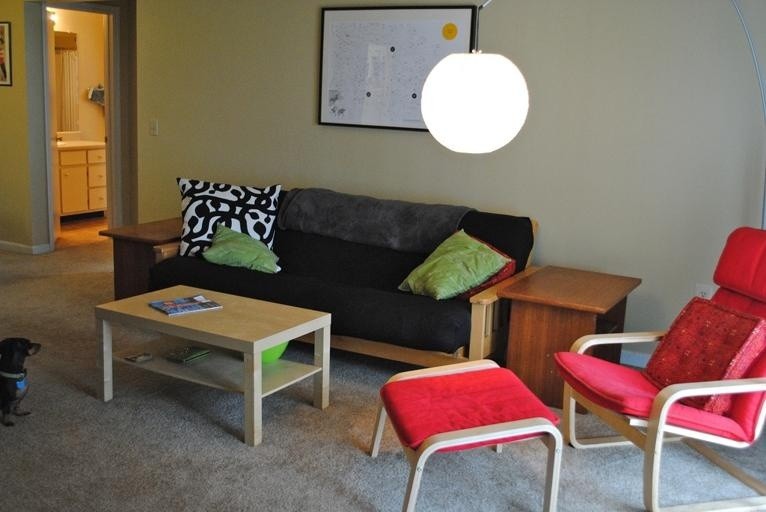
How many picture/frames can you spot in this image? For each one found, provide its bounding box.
[0,21,12,88]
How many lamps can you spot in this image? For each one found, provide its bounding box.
[419,0,532,156]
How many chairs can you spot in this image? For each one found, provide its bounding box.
[548,224,766,511]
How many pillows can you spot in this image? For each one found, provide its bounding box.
[462,235,518,298]
[172,172,284,258]
[201,221,281,276]
[397,227,514,304]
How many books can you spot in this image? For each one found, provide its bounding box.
[160,345,212,365]
[146,293,223,318]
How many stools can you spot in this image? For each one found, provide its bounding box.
[368,357,565,512]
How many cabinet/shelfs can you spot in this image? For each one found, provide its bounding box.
[497,264,643,416]
[58,147,88,215]
[89,148,107,213]
[97,216,184,302]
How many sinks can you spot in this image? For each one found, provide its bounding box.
[58,141,65,146]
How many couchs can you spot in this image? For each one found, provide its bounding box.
[145,187,538,366]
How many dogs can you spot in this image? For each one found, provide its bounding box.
[0,338,40,426]
